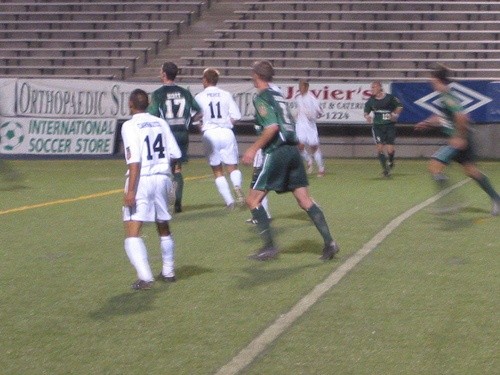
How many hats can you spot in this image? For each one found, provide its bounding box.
[250,60,275,77]
[425,62,454,84]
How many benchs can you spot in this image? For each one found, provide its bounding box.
[0,0,500,82]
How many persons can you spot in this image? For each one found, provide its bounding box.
[190,67,247,211]
[364,81,405,176]
[120,88,182,289]
[241,60,339,261]
[415,62,500,216]
[146,62,204,213]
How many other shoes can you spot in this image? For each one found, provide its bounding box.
[130,279,156,290]
[173,201,182,213]
[305,160,314,175]
[224,201,238,211]
[246,215,273,225]
[316,167,324,176]
[491,194,500,217]
[244,245,280,261]
[383,162,394,177]
[318,240,339,262]
[155,271,177,283]
[236,198,248,208]
